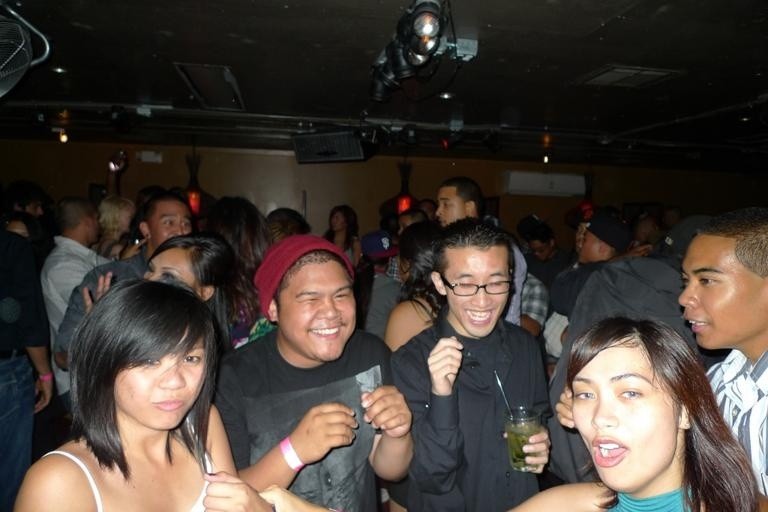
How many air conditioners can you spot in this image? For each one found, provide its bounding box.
[502,169,587,201]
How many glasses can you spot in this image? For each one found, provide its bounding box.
[439,272,512,297]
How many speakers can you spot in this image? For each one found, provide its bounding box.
[290,130,372,164]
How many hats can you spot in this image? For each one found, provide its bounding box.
[585,213,627,250]
[252,234,355,322]
[360,229,400,257]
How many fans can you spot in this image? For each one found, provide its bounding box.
[0,0,52,103]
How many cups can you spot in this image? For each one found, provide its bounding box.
[502,405,546,473]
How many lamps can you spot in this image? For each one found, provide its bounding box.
[361,0,450,103]
